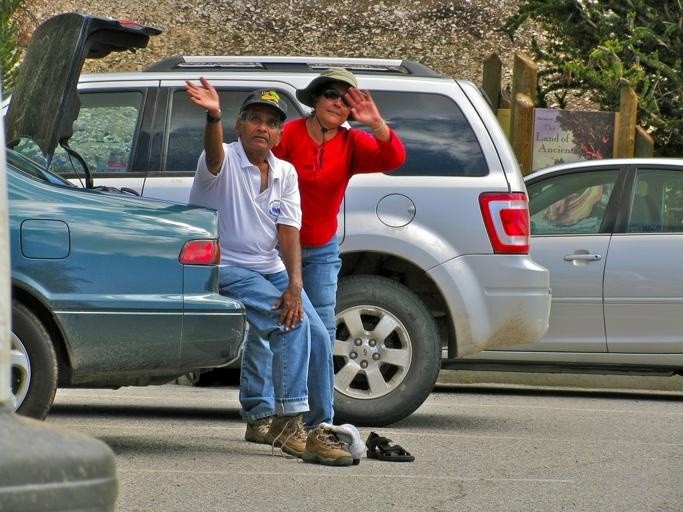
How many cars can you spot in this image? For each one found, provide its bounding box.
[1,13,252,423]
[435,157,683,378]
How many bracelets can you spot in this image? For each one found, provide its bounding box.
[206,108,223,122]
[372,118,384,132]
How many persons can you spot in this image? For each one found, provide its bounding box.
[239,66,407,443]
[184,77,333,457]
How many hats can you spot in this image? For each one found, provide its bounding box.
[295,67,366,122]
[239,89,288,122]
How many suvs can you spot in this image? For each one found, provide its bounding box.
[1,55,553,427]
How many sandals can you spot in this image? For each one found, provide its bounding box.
[366,432,416,461]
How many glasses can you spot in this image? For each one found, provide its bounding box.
[321,87,350,107]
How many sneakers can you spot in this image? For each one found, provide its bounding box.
[302,429,353,466]
[244,418,271,443]
[263,412,306,458]
